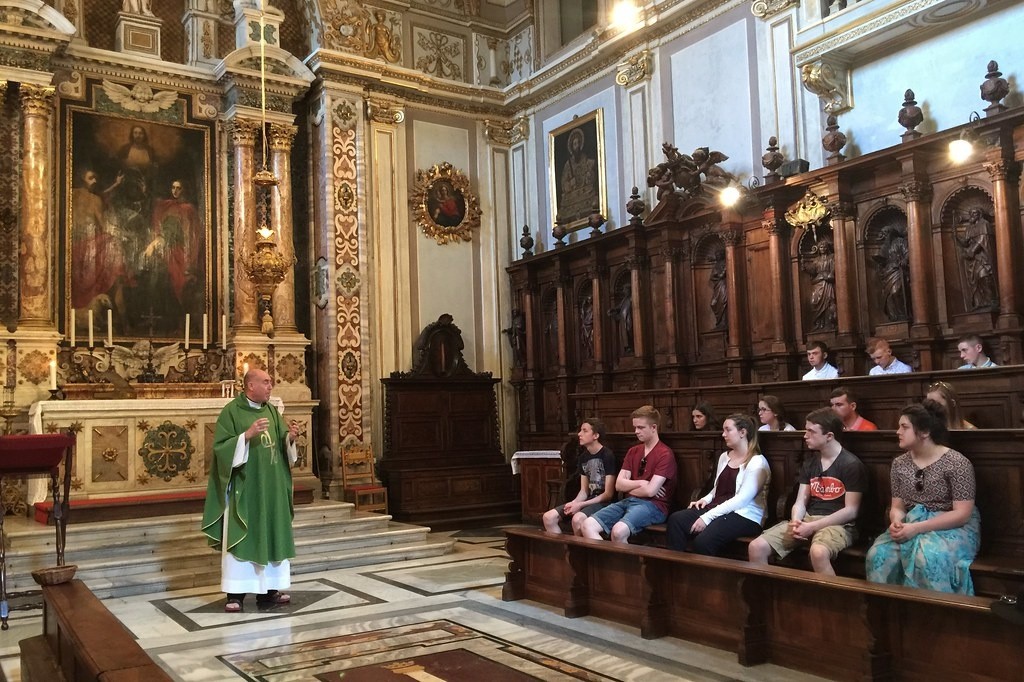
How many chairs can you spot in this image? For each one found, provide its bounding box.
[340,439,389,514]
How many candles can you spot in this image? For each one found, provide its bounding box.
[185,313,190,349]
[107,309,112,345]
[222,315,226,350]
[88,309,93,347]
[49,359,57,390]
[203,314,208,349]
[70,308,76,347]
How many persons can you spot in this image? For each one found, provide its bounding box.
[680,147,730,188]
[372,9,400,62]
[803,341,838,379]
[608,282,633,352]
[542,417,619,537]
[867,400,981,596]
[801,241,837,329]
[502,306,525,367]
[951,207,1000,309]
[956,333,998,370]
[925,381,978,430]
[865,338,912,375]
[708,250,728,330]
[756,395,797,431]
[201,368,299,611]
[545,300,559,362]
[581,405,677,544]
[580,296,595,359]
[691,400,720,431]
[829,386,878,431]
[748,408,870,578]
[872,225,909,320]
[664,413,772,557]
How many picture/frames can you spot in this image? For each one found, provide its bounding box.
[409,160,483,248]
[547,107,608,234]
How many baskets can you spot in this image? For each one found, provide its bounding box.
[31,565,77,585]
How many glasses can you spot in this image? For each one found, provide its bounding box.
[756,407,773,413]
[638,458,647,477]
[914,469,925,492]
[928,381,948,389]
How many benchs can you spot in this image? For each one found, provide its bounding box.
[501,364,1024,601]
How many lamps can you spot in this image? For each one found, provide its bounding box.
[947,110,988,168]
[719,175,762,214]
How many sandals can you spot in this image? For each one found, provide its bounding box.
[225,593,246,610]
[256,590,290,604]
